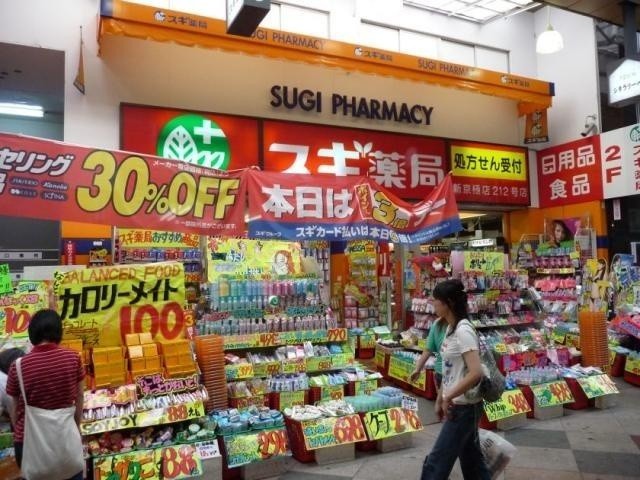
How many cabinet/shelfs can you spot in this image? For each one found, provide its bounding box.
[405,237,600,360]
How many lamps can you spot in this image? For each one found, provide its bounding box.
[534,5,564,54]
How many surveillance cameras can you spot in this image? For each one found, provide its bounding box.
[581,123,595,137]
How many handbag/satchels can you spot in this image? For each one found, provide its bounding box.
[457,322,506,401]
[478,427,516,480]
[15,357,83,480]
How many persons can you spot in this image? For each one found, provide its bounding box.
[420,278,487,480]
[5,309,86,480]
[610,255,623,272]
[409,317,449,389]
[0,346,29,427]
[547,219,575,247]
[586,257,608,313]
